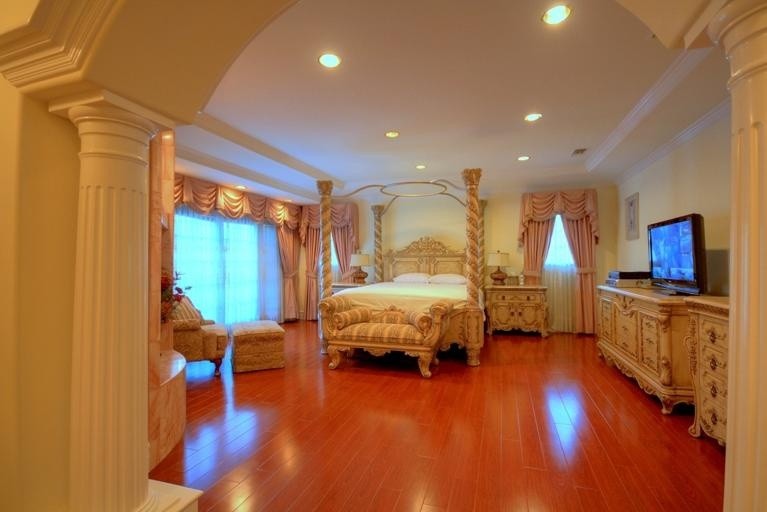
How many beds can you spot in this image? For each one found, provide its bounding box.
[317,168,488,366]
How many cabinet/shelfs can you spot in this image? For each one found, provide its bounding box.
[684,296,729,448]
[486,285,550,339]
[595,283,694,415]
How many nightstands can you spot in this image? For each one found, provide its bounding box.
[317,283,364,296]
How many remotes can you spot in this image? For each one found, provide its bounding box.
[659,288,677,295]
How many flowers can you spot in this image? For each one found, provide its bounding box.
[161,269,192,324]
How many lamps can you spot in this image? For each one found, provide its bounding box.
[487,250,510,285]
[350,250,370,283]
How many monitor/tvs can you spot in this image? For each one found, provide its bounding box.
[647,213,708,296]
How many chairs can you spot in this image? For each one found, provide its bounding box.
[174,295,228,377]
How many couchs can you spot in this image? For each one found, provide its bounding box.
[318,294,454,378]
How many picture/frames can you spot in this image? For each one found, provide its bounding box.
[625,192,640,241]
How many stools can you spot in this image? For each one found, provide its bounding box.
[231,320,286,373]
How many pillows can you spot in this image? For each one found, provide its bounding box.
[171,298,202,326]
